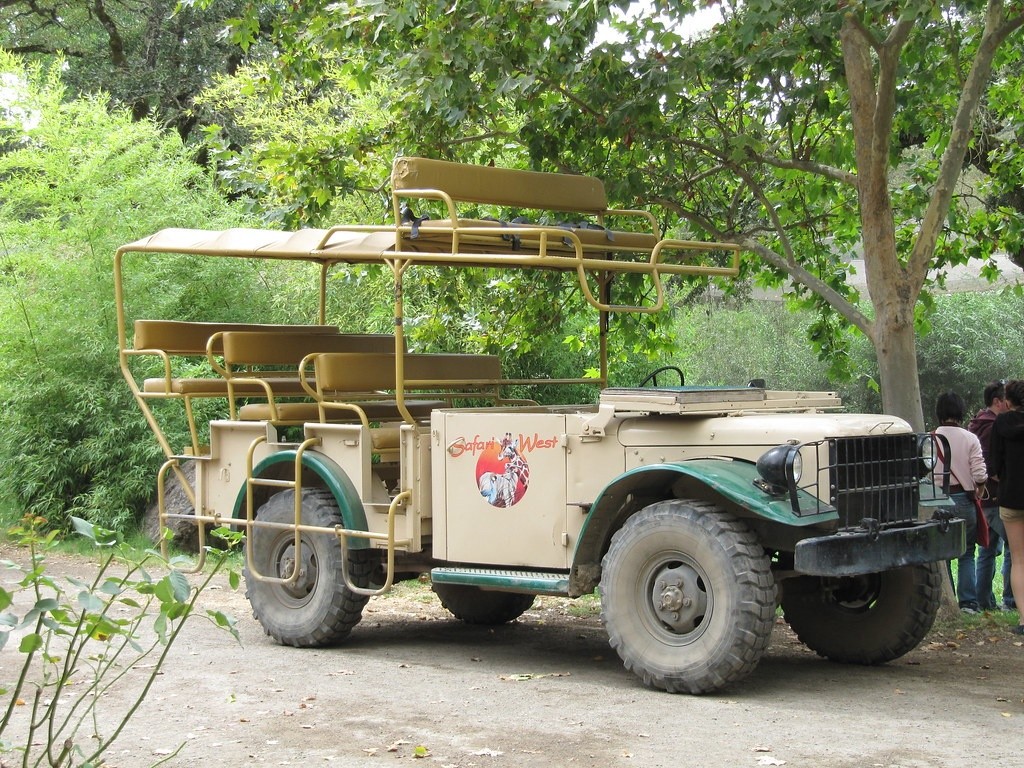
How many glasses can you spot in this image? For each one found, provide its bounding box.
[991,379,1006,394]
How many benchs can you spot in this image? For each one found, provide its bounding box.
[206,331,453,426]
[391,156,667,252]
[297,353,540,463]
[132,320,387,397]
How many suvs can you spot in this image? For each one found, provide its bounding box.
[115,153,968,698]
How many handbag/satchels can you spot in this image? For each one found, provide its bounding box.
[975,499,989,548]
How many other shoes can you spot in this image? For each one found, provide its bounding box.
[1011,623,1024,635]
[1002,604,1014,610]
[958,601,980,614]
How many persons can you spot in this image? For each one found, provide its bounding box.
[968,382,1018,612]
[990,380,1024,636]
[928,392,988,614]
